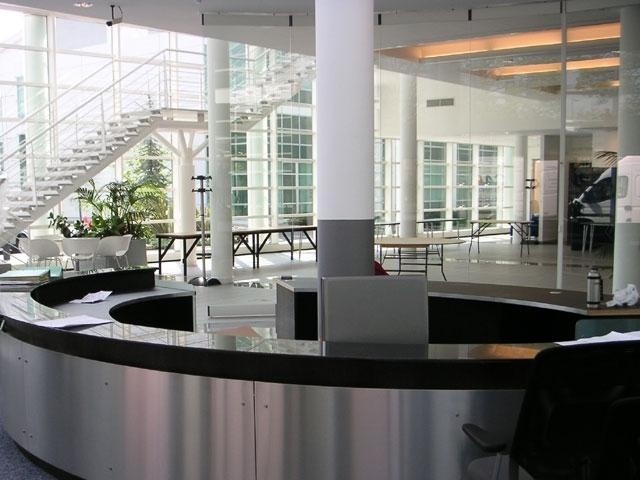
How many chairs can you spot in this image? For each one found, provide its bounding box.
[20,234,132,275]
[460,338,640,477]
[320,274,432,345]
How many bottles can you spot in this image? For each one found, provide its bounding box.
[586,266,604,309]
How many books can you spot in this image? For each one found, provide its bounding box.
[1,268,51,286]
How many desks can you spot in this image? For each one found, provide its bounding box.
[155,224,317,278]
[373,216,535,282]
[580,221,613,254]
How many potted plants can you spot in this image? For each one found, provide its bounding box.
[49,175,147,272]
